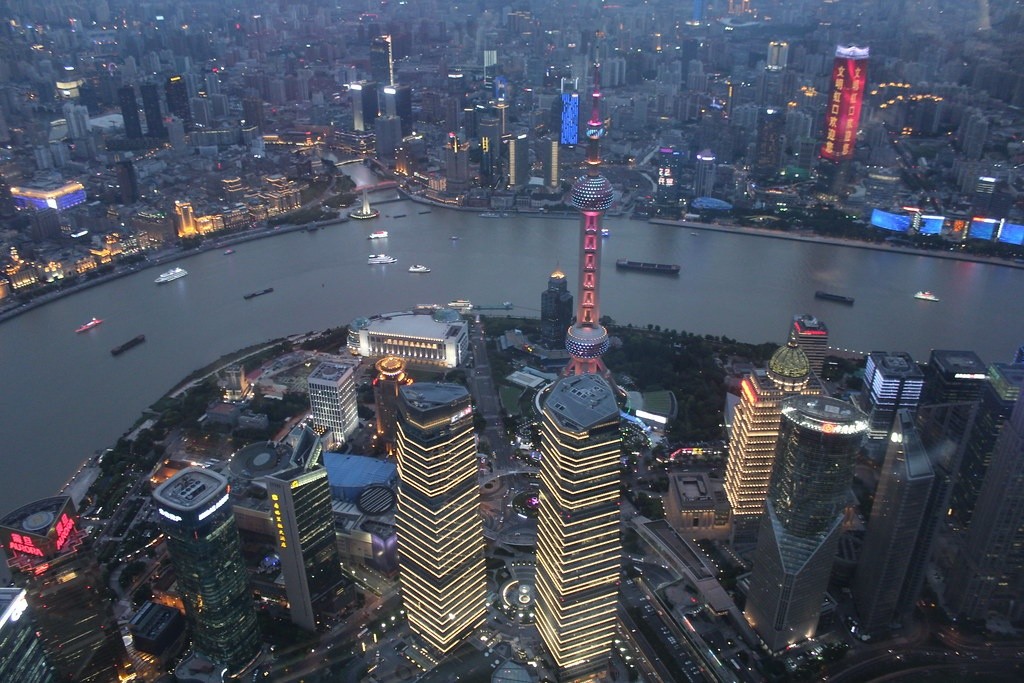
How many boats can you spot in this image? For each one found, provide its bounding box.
[370,231,388,239]
[223,249,235,255]
[73,317,103,333]
[368,254,397,264]
[243,287,273,299]
[408,265,431,273]
[154,267,188,283]
[815,291,855,304]
[616,258,681,274]
[111,334,145,355]
[602,229,608,236]
[914,290,939,302]
[478,212,508,218]
